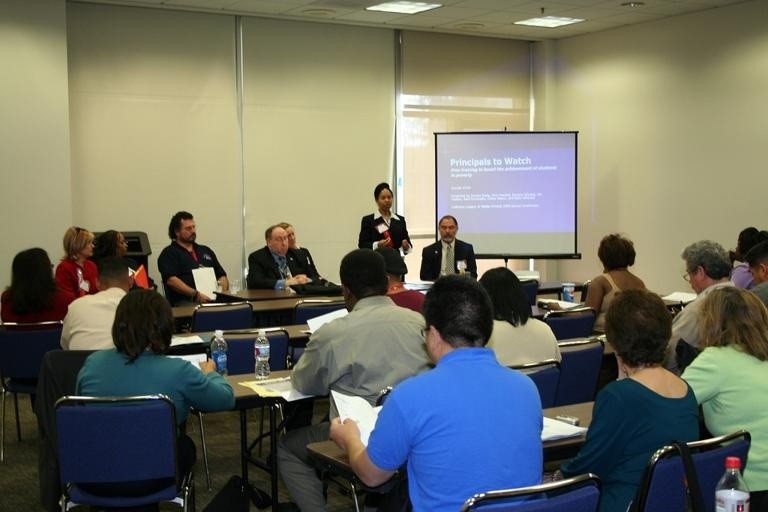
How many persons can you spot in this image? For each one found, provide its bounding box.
[60,252,129,350]
[247,224,312,290]
[420,215,478,281]
[93,230,158,293]
[679,285,768,512]
[745,240,768,308]
[158,211,229,308]
[55,226,99,299]
[662,240,736,375]
[0,247,74,414]
[277,222,339,287]
[730,227,767,291]
[374,245,426,314]
[277,249,436,512]
[358,182,413,282]
[478,267,562,373]
[584,233,646,336]
[75,289,236,512]
[330,274,543,512]
[544,288,699,512]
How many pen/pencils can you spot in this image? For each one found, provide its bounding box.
[206,348,210,361]
[680,301,684,310]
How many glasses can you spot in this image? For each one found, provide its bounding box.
[682,271,691,282]
[420,326,431,338]
[119,240,128,245]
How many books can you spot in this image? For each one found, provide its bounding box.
[128,266,149,289]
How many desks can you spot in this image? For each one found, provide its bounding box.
[538,282,586,302]
[557,335,615,355]
[306,401,594,512]
[162,280,430,317]
[190,371,321,512]
[172,326,311,347]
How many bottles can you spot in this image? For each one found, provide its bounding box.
[716,457,750,512]
[211,330,227,378]
[255,330,270,379]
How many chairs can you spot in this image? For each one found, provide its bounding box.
[190,301,254,327]
[557,338,605,405]
[457,472,600,512]
[194,330,289,489]
[543,307,597,339]
[294,300,347,323]
[625,430,751,511]
[512,361,562,407]
[52,395,193,512]
[0,322,80,461]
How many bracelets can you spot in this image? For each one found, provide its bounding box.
[190,289,200,303]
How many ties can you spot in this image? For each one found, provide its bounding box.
[446,246,454,276]
[280,257,289,279]
